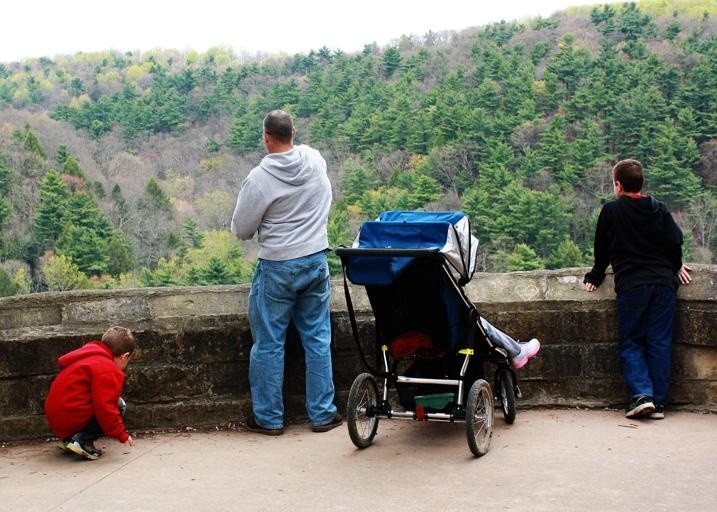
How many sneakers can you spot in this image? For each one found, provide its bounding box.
[57,436,102,460]
[245,417,283,436]
[512,338,540,367]
[626,397,665,421]
[312,413,342,431]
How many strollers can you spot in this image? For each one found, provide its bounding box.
[335,211,521,456]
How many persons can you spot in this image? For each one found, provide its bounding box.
[43,326,137,460]
[231,109,343,436]
[584,159,693,420]
[480,315,540,369]
